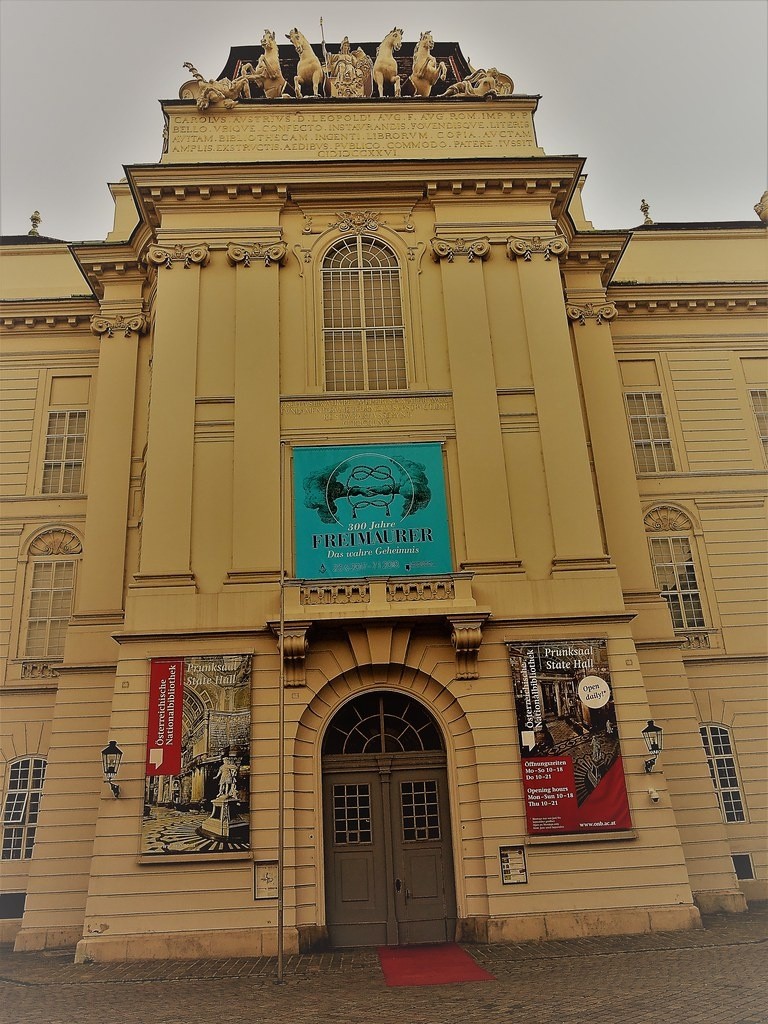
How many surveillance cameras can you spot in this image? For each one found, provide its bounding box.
[647,788,660,805]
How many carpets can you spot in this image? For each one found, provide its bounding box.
[378,943,499,987]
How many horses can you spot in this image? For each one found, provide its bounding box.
[240,29,287,97]
[284,27,323,98]
[408,31,447,98]
[373,27,405,98]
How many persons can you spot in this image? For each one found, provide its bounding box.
[436,65,500,102]
[182,61,267,111]
[212,757,238,797]
[321,35,364,86]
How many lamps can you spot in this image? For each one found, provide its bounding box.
[641,719,664,773]
[99,740,123,798]
